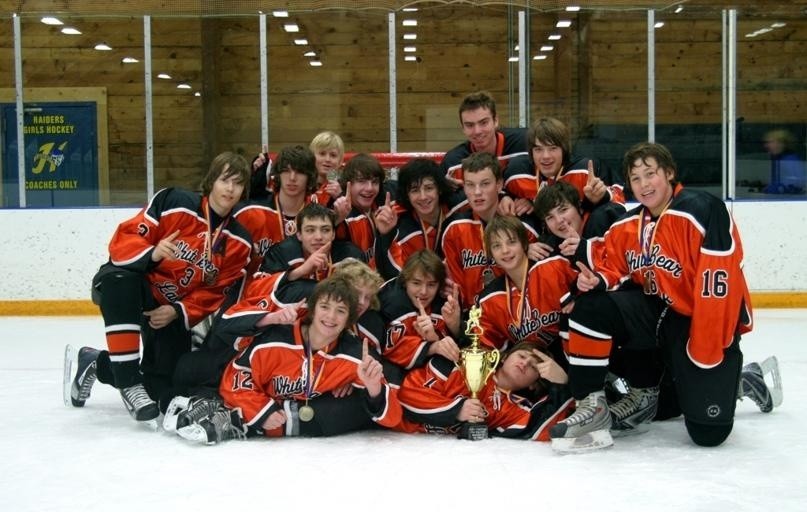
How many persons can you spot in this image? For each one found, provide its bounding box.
[374,251,460,356]
[161,258,384,414]
[439,91,538,216]
[198,203,367,345]
[250,132,346,208]
[222,277,403,433]
[504,117,624,212]
[749,123,807,194]
[164,338,570,446]
[432,152,541,311]
[375,157,468,280]
[549,145,754,454]
[64,154,254,426]
[527,182,627,270]
[471,216,784,414]
[326,153,403,267]
[258,146,350,243]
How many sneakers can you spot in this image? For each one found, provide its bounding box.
[737,357,773,413]
[121,384,159,422]
[71,345,102,408]
[176,395,225,431]
[607,387,659,429]
[549,392,613,439]
[198,410,244,446]
